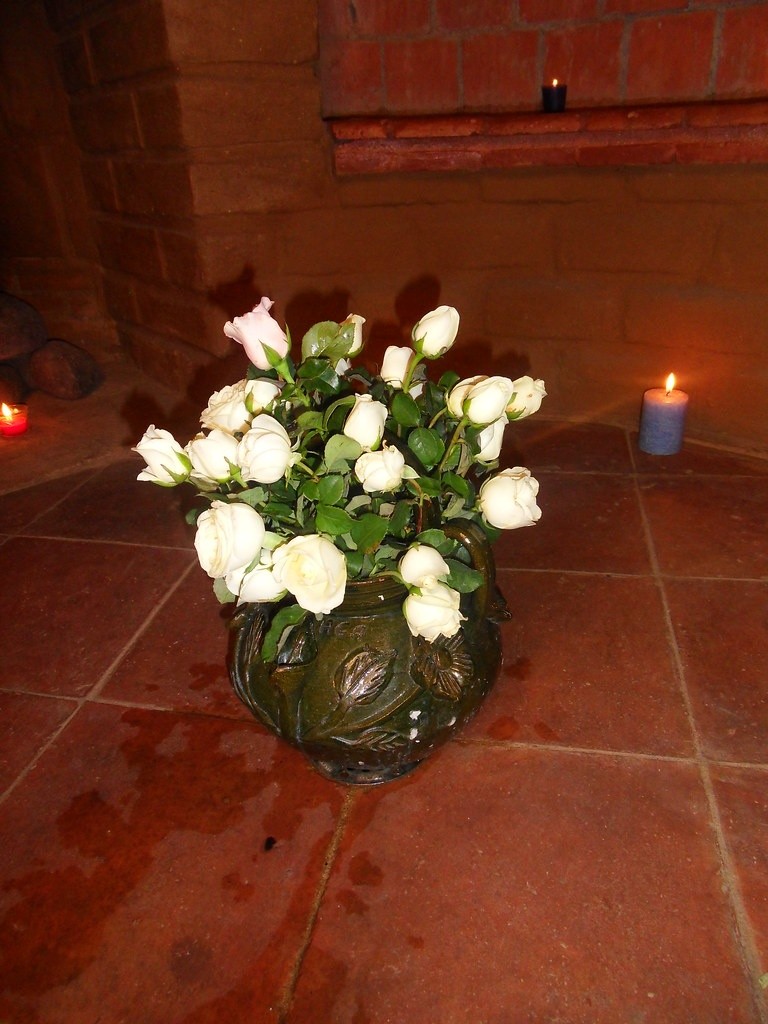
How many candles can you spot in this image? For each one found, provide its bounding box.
[541,78,566,114]
[0,403,30,438]
[639,372,690,456]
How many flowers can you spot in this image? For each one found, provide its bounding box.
[130,294,547,663]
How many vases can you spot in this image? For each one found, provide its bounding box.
[226,498,513,786]
